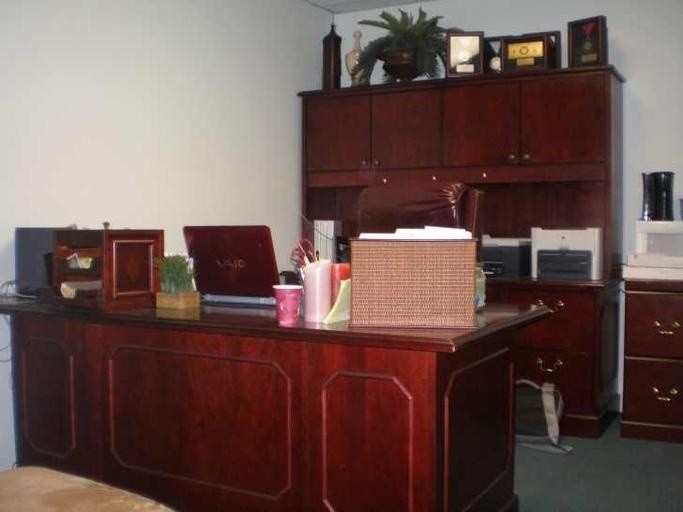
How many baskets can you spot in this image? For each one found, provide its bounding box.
[349,237,477,329]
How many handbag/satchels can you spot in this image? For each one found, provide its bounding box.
[514,379,574,454]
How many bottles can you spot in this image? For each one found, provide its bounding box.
[321,23,342,90]
[345,30,366,88]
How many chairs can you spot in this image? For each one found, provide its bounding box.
[353,169,486,239]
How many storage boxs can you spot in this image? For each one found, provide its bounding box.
[524,222,605,282]
[472,229,532,280]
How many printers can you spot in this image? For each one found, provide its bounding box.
[531,226,604,282]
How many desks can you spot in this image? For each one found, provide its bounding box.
[5,295,552,512]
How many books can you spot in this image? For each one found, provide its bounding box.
[322,277,352,324]
[313,220,342,262]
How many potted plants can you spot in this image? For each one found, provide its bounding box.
[147,254,204,309]
[348,3,454,82]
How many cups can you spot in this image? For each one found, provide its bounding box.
[271,284,301,330]
[331,262,350,307]
[639,171,675,221]
[302,259,333,323]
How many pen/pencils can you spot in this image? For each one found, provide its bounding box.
[289,241,320,270]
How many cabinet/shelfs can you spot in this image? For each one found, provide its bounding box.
[616,276,683,441]
[439,65,628,170]
[293,80,441,175]
[499,279,619,440]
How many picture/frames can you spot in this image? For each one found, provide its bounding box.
[102,228,164,313]
[566,15,604,70]
[446,31,484,77]
[523,30,561,71]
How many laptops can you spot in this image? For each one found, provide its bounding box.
[183,225,283,306]
